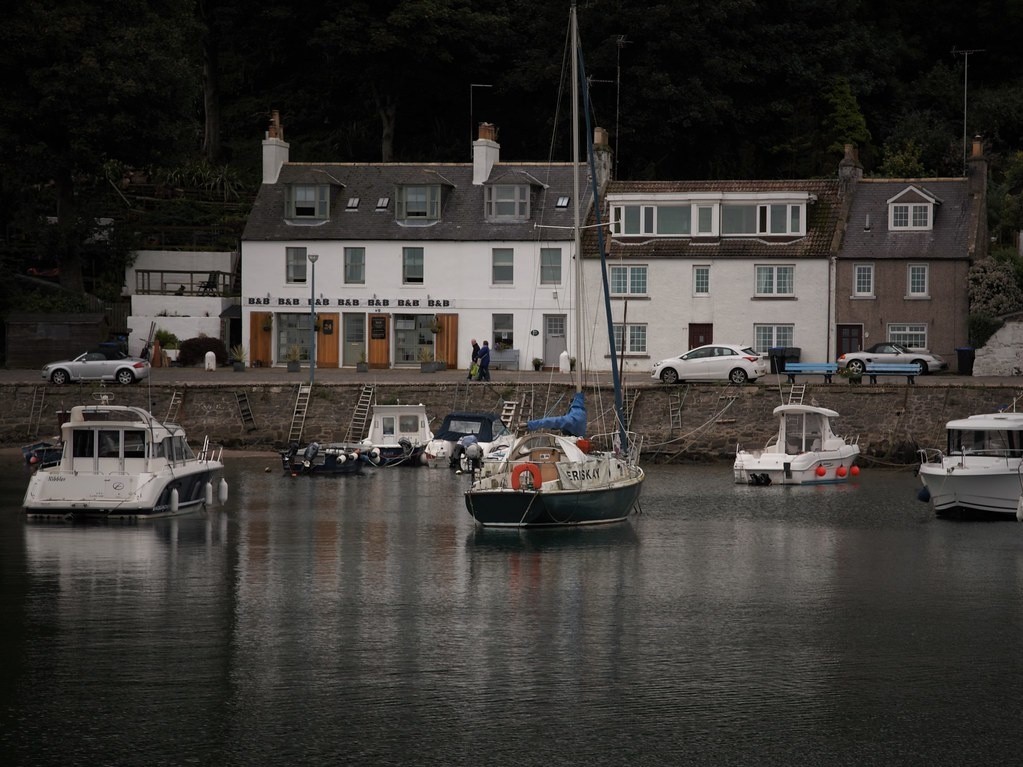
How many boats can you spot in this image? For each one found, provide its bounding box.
[733,356,861,486]
[917,394,1023,522]
[20,434,64,472]
[23,361,227,521]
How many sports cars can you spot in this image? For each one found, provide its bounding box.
[837,342,950,376]
[40,348,152,386]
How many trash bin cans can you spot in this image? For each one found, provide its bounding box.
[955,348,975,374]
[98,343,121,360]
[769,347,802,374]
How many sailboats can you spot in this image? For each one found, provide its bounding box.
[281,0,646,530]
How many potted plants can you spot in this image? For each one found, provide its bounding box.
[357,354,369,372]
[263,321,272,331]
[532,358,541,371]
[840,367,861,385]
[156,329,182,362]
[430,322,441,333]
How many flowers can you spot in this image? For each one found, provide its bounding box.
[494,342,509,350]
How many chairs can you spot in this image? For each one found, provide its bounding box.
[196,270,221,296]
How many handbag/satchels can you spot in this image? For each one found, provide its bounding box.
[477,358,481,365]
[471,364,478,376]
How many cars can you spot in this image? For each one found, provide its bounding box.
[650,343,768,385]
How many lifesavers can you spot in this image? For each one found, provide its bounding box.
[511,463,542,490]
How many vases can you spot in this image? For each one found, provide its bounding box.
[315,325,321,331]
[421,361,447,372]
[233,362,245,372]
[287,361,300,372]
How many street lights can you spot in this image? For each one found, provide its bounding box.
[307,254,319,381]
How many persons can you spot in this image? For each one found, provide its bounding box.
[475,341,490,381]
[467,339,482,381]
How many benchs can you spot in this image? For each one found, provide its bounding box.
[862,363,922,384]
[489,350,520,370]
[781,363,838,383]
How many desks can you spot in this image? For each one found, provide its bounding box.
[163,282,197,295]
